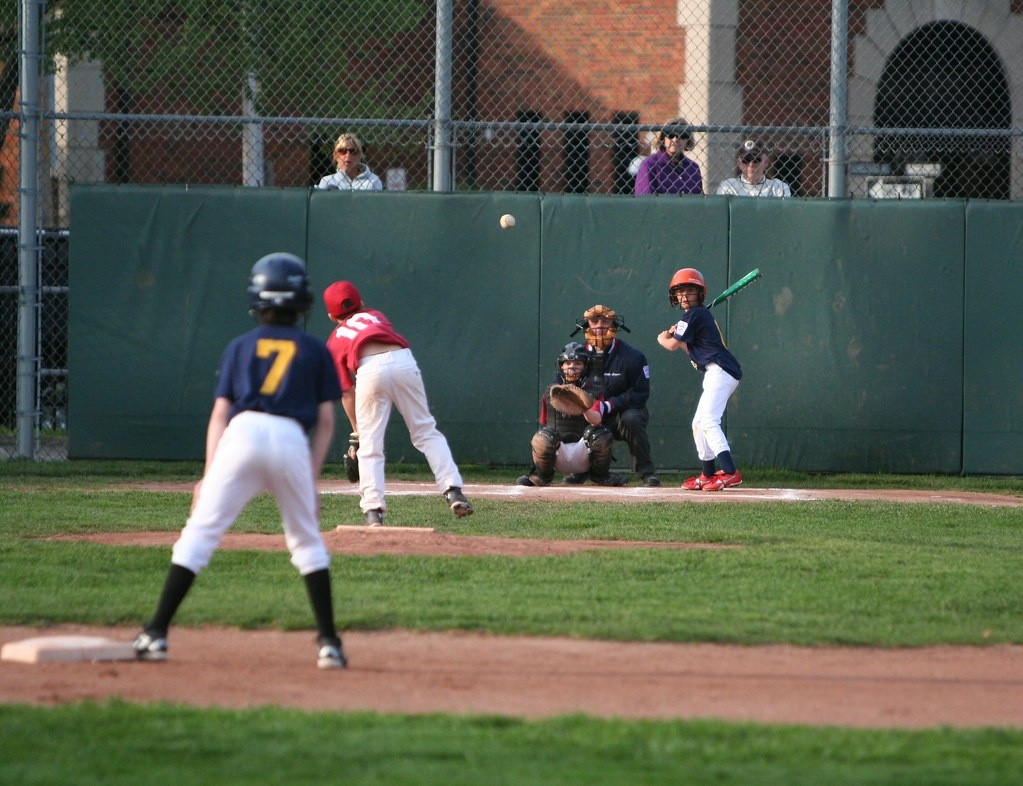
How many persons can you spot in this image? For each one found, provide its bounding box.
[717,132,790,197]
[131,252,349,669]
[323,280,476,527]
[529,342,628,485]
[635,116,702,194]
[515,305,665,487]
[318,133,382,190]
[656,268,744,492]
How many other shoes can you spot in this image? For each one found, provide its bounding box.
[641,473,661,487]
[598,472,628,485]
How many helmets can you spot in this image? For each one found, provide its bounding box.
[246,251,312,312]
[556,342,590,368]
[582,303,616,352]
[668,268,707,306]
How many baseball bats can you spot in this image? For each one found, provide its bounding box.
[705,267,764,311]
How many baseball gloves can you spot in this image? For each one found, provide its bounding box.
[548,383,591,417]
[343,433,360,483]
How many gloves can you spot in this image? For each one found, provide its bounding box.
[592,401,611,417]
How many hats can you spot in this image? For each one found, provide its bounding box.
[734,132,770,158]
[324,281,363,317]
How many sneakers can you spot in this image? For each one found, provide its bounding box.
[561,471,589,486]
[517,464,555,486]
[681,473,711,490]
[132,622,169,660]
[365,508,383,527]
[315,635,348,670]
[703,468,743,491]
[443,488,474,520]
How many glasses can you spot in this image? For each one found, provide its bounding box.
[666,132,688,140]
[337,147,360,156]
[741,156,762,164]
